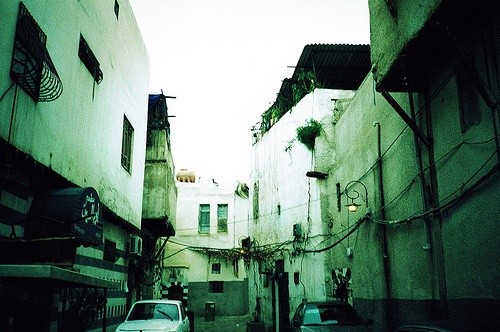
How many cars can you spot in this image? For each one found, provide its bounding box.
[289,300,371,332]
[114,299,191,332]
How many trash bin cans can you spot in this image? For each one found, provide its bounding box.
[204,301,216,321]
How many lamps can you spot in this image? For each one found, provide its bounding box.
[344,180,369,216]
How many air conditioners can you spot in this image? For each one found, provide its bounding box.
[127,235,143,256]
